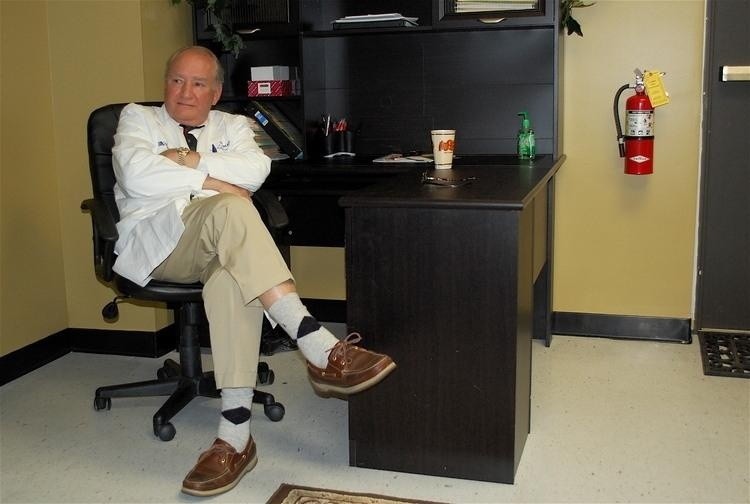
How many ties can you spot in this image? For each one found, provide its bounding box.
[180,123,205,152]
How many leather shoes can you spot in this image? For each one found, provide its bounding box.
[181,432,258,497]
[306,338,397,398]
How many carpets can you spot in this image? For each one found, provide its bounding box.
[698,329,750,380]
[265,483,450,503]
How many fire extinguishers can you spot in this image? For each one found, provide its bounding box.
[614,67,668,175]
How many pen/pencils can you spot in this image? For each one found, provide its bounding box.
[321,112,351,136]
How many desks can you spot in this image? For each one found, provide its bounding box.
[338,156,567,484]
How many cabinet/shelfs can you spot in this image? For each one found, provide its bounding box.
[191,0,563,167]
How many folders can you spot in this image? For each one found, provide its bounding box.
[243,101,303,160]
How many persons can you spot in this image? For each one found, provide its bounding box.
[107,43,399,497]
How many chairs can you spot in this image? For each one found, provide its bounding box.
[80,102,293,441]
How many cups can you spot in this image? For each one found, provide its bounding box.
[430,130,456,169]
[320,130,356,155]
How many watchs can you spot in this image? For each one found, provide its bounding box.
[176,145,191,164]
